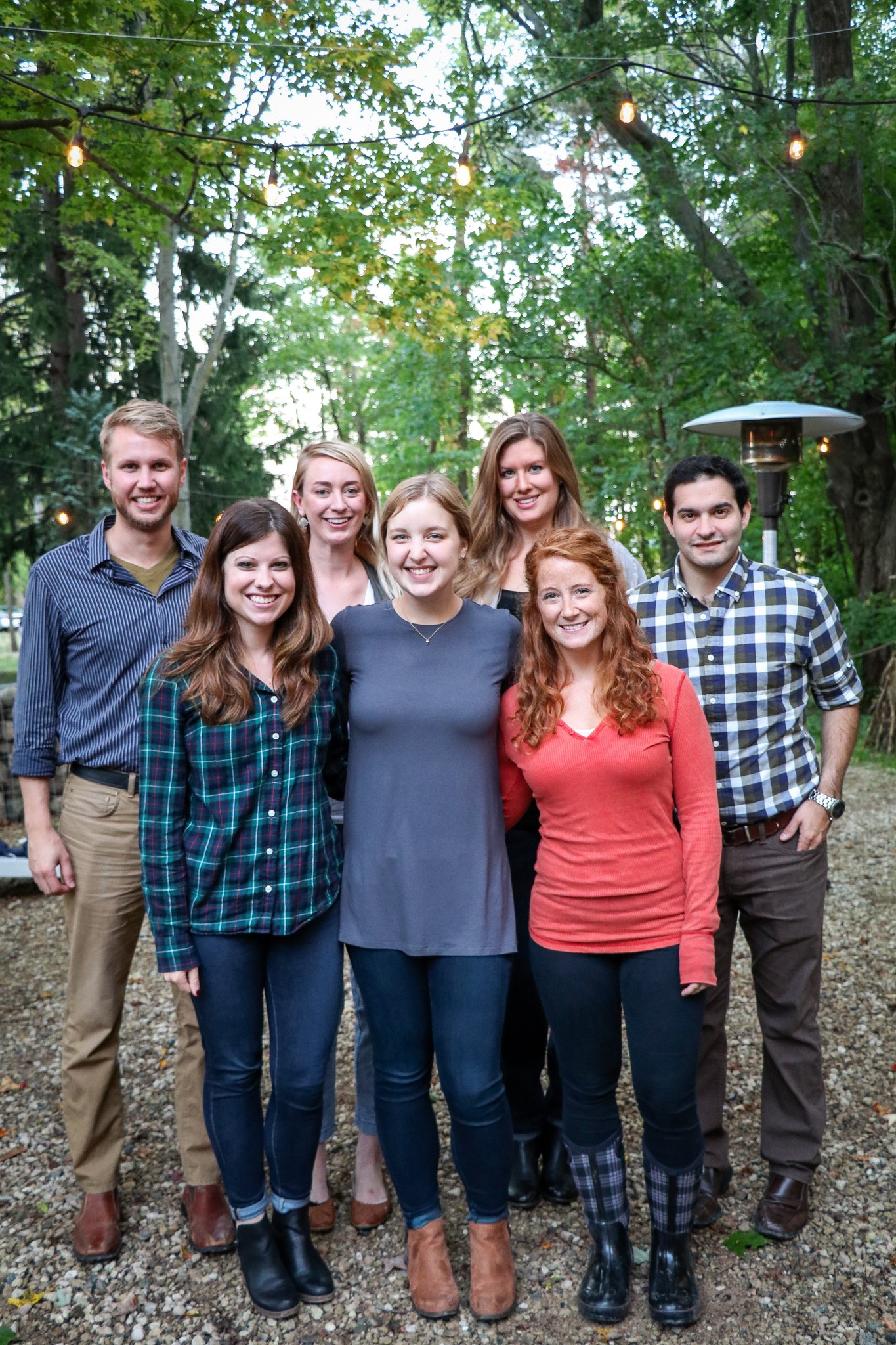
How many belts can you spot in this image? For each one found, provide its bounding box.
[722,807,798,846]
[69,762,140,795]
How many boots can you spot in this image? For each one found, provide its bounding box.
[642,1132,701,1325]
[563,1122,633,1323]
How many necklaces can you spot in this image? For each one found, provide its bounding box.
[404,612,447,644]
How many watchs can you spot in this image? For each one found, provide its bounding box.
[809,790,845,818]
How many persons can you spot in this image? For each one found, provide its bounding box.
[288,440,404,1231]
[625,456,863,1242]
[496,527,723,1325]
[9,400,236,1263]
[448,414,646,1208]
[137,497,353,1320]
[313,473,523,1322]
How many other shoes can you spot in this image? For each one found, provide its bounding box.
[407,1216,458,1318]
[468,1219,516,1322]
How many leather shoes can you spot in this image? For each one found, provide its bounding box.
[507,1135,542,1208]
[180,1183,235,1256]
[269,1203,335,1303]
[543,1132,579,1206]
[350,1171,391,1236]
[693,1160,732,1225]
[72,1188,122,1263]
[756,1170,808,1240]
[308,1197,335,1232]
[233,1208,301,1319]
